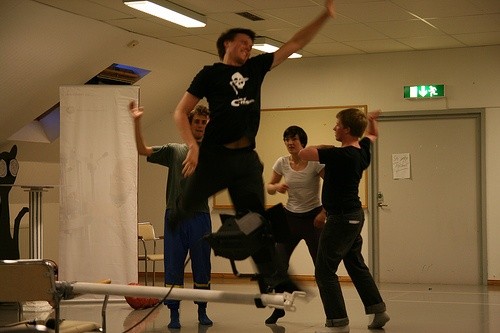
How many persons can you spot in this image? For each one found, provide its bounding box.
[297,106,391,333]
[174,0,335,294]
[265,124,327,324]
[127,98,214,329]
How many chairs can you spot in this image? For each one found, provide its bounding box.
[138,222,191,286]
[0,258,112,333]
